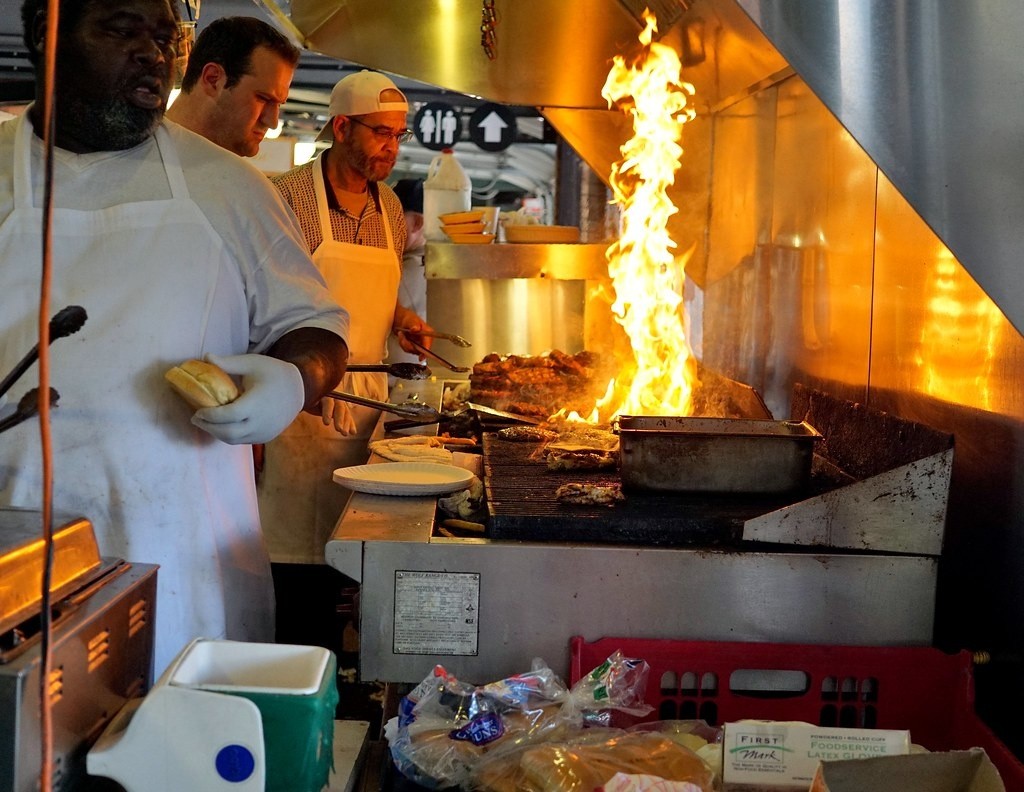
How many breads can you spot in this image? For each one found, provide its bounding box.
[404,703,718,791]
[164,359,239,409]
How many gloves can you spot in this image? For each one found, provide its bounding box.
[188,351,305,445]
[308,395,357,436]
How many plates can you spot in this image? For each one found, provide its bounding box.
[437,210,495,244]
[333,463,476,496]
[505,224,581,244]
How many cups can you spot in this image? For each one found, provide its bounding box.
[472,206,500,243]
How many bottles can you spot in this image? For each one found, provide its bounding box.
[422,149,471,242]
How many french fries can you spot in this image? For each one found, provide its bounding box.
[435,436,476,444]
[443,519,485,532]
[438,527,454,536]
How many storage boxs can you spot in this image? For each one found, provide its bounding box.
[569,633,1024,791]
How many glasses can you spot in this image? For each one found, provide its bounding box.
[350,116,413,144]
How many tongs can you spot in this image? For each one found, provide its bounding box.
[390,326,471,374]
[0,305,88,432]
[322,362,441,423]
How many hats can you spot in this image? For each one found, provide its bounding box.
[391,178,425,213]
[314,69,408,142]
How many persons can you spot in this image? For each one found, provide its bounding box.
[0,0,351,683]
[268,69,434,653]
[382,177,427,387]
[165,16,356,484]
